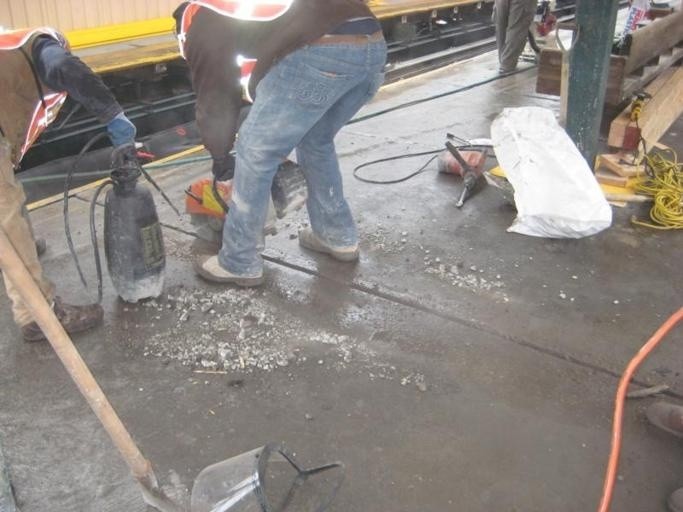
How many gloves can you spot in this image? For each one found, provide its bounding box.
[211,155,236,182]
[107,111,138,149]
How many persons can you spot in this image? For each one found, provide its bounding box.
[495,0,537,74]
[172,0,388,287]
[1,27,137,341]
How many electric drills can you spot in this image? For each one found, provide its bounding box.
[438,141,489,208]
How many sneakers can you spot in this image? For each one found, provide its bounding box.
[298,226,360,263]
[191,253,265,288]
[21,296,105,342]
